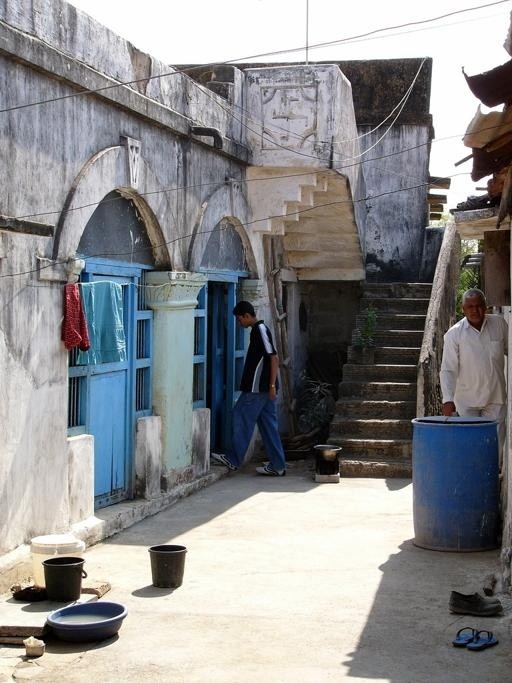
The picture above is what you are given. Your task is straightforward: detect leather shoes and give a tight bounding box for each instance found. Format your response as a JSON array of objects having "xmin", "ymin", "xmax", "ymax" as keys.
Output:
[
  {"xmin": 450, "ymin": 591, "xmax": 500, "ymax": 605},
  {"xmin": 449, "ymin": 598, "xmax": 502, "ymax": 617}
]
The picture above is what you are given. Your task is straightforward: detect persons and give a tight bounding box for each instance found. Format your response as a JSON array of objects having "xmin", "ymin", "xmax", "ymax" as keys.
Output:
[
  {"xmin": 212, "ymin": 300, "xmax": 286, "ymax": 476},
  {"xmin": 439, "ymin": 287, "xmax": 508, "ymax": 473},
  {"xmin": 307, "ymin": 343, "xmax": 345, "ymax": 382}
]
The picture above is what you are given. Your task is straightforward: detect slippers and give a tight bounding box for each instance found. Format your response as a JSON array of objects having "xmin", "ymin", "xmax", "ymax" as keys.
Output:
[
  {"xmin": 466, "ymin": 630, "xmax": 499, "ymax": 651},
  {"xmin": 451, "ymin": 626, "xmax": 479, "ymax": 646}
]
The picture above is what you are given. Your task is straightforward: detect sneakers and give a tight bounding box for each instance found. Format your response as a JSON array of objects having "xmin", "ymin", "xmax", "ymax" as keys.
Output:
[
  {"xmin": 211, "ymin": 452, "xmax": 239, "ymax": 471},
  {"xmin": 255, "ymin": 464, "xmax": 286, "ymax": 477}
]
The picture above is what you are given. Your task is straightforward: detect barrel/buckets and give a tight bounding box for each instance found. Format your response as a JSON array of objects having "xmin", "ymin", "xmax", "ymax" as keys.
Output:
[
  {"xmin": 313, "ymin": 445, "xmax": 342, "ymax": 475},
  {"xmin": 412, "ymin": 415, "xmax": 500, "ymax": 553},
  {"xmin": 30, "ymin": 535, "xmax": 86, "ymax": 589},
  {"xmin": 41, "ymin": 557, "xmax": 85, "ymax": 602},
  {"xmin": 148, "ymin": 544, "xmax": 186, "ymax": 588}
]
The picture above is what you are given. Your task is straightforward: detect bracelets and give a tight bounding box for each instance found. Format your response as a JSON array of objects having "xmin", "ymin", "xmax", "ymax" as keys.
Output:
[{"xmin": 269, "ymin": 383, "xmax": 275, "ymax": 387}]
